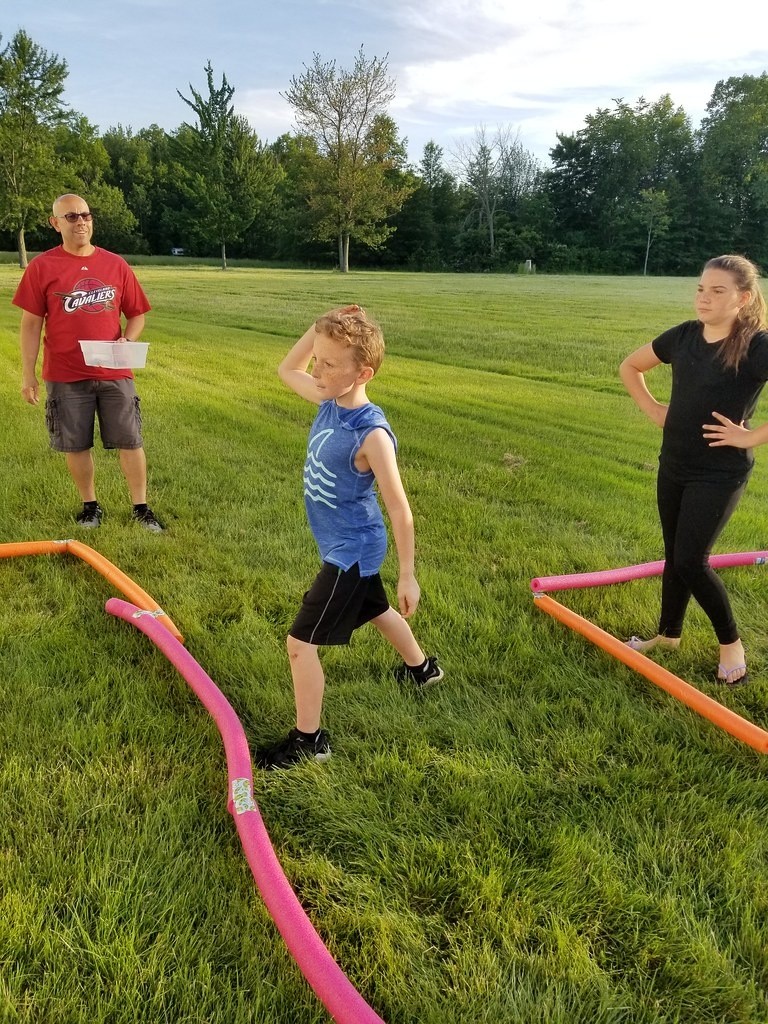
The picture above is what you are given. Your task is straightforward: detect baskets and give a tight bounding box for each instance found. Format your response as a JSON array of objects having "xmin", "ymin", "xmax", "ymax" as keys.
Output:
[{"xmin": 79, "ymin": 340, "xmax": 149, "ymax": 369}]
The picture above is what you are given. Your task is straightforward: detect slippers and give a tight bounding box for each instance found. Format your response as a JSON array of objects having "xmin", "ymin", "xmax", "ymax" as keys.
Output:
[
  {"xmin": 716, "ymin": 655, "xmax": 747, "ymax": 687},
  {"xmin": 628, "ymin": 636, "xmax": 644, "ymax": 651}
]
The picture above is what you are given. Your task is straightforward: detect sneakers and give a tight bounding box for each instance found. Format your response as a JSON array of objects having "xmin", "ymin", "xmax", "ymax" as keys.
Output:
[
  {"xmin": 132, "ymin": 509, "xmax": 163, "ymax": 533},
  {"xmin": 403, "ymin": 656, "xmax": 444, "ymax": 690},
  {"xmin": 76, "ymin": 506, "xmax": 102, "ymax": 528},
  {"xmin": 253, "ymin": 729, "xmax": 331, "ymax": 773}
]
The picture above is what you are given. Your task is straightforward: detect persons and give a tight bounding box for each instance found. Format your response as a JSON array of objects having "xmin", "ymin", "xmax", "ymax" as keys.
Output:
[
  {"xmin": 618, "ymin": 253, "xmax": 767, "ymax": 686},
  {"xmin": 13, "ymin": 194, "xmax": 166, "ymax": 536},
  {"xmin": 255, "ymin": 306, "xmax": 444, "ymax": 772}
]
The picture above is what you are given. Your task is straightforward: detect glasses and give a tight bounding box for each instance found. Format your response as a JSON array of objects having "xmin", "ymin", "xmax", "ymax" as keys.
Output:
[{"xmin": 56, "ymin": 213, "xmax": 94, "ymax": 223}]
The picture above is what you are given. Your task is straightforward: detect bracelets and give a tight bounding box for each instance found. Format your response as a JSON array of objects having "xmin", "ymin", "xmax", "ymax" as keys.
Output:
[{"xmin": 126, "ymin": 338, "xmax": 131, "ymax": 342}]
